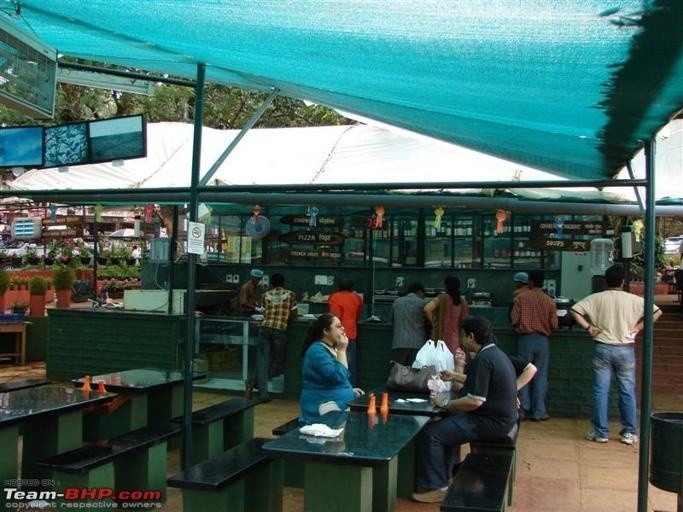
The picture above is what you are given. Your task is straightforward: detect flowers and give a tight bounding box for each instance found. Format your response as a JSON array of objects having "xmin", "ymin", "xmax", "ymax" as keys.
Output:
[{"xmin": 60, "ymin": 242, "xmax": 72, "ymax": 261}]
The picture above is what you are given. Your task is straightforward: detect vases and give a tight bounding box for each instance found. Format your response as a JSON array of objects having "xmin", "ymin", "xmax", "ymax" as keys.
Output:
[{"xmin": 59, "ymin": 257, "xmax": 72, "ymax": 265}]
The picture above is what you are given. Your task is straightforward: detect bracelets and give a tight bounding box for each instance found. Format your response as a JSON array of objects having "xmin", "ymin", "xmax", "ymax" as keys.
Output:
[{"xmin": 585, "ymin": 324, "xmax": 591, "ymax": 330}]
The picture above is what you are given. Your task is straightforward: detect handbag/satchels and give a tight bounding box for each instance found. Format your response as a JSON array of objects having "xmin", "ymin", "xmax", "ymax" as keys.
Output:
[
  {"xmin": 385, "ymin": 360, "xmax": 437, "ymax": 396},
  {"xmin": 430, "ymin": 295, "xmax": 446, "ymax": 344}
]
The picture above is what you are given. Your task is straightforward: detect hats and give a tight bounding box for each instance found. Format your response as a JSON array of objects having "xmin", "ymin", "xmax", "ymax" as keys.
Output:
[
  {"xmin": 250, "ymin": 269, "xmax": 264, "ymax": 278},
  {"xmin": 514, "ymin": 272, "xmax": 529, "ymax": 283}
]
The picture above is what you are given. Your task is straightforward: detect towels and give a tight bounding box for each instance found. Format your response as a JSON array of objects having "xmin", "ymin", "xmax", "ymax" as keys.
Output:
[{"xmin": 300, "ymin": 423, "xmax": 343, "ymax": 437}]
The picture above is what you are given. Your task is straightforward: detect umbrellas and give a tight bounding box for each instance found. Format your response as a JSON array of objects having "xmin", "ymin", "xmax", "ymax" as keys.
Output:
[{"xmin": 109, "ymin": 228, "xmax": 144, "ymax": 241}]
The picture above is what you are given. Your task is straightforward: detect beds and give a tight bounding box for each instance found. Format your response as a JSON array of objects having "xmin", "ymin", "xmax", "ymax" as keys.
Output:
[
  {"xmin": 346, "ymin": 381, "xmax": 444, "ymax": 500},
  {"xmin": 72, "ymin": 367, "xmax": 207, "ymax": 443}
]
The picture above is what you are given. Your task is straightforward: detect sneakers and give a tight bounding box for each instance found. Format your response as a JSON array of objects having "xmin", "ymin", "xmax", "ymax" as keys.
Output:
[
  {"xmin": 620, "ymin": 433, "xmax": 639, "ymax": 444},
  {"xmin": 245, "ymin": 378, "xmax": 254, "ymax": 400},
  {"xmin": 411, "ymin": 490, "xmax": 448, "ymax": 503},
  {"xmin": 584, "ymin": 430, "xmax": 608, "ymax": 442},
  {"xmin": 519, "ymin": 412, "xmax": 528, "ymax": 420},
  {"xmin": 529, "ymin": 414, "xmax": 550, "ymax": 422},
  {"xmin": 446, "ymin": 479, "xmax": 453, "ymax": 487},
  {"xmin": 258, "ymin": 396, "xmax": 273, "ymax": 404}
]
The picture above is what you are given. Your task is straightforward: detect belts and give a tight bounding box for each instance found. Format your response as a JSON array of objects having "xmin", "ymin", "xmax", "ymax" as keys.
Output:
[
  {"xmin": 592, "ymin": 340, "xmax": 634, "ymax": 347},
  {"xmin": 521, "ymin": 332, "xmax": 544, "ymax": 335}
]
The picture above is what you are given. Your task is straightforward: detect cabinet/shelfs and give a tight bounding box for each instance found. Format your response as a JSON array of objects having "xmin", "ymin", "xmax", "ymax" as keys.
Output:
[{"xmin": 285, "ymin": 214, "xmax": 566, "ymax": 273}]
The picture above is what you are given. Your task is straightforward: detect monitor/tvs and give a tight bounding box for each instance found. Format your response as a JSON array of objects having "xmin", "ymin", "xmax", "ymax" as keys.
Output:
[
  {"xmin": 0, "ymin": 126, "xmax": 46, "ymax": 168},
  {"xmin": 38, "ymin": 121, "xmax": 88, "ymax": 169},
  {"xmin": 88, "ymin": 114, "xmax": 147, "ymax": 163}
]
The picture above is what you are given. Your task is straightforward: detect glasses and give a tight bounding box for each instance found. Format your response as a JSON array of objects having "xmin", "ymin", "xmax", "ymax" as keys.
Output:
[{"xmin": 254, "ymin": 278, "xmax": 260, "ymax": 281}]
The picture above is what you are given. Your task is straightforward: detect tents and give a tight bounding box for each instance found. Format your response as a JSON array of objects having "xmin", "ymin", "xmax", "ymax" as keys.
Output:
[
  {"xmin": 3, "ymin": 118, "xmax": 683, "ymax": 299},
  {"xmin": 0, "ymin": 2, "xmax": 682, "ymax": 512}
]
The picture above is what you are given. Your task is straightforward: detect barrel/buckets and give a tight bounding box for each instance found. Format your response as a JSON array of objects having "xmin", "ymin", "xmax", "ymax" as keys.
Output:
[{"xmin": 590, "ymin": 238, "xmax": 614, "ymax": 275}]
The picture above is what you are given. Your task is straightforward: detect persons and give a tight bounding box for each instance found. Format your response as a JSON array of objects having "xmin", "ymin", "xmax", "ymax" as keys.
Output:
[
  {"xmin": 512, "ymin": 272, "xmax": 529, "ymax": 299},
  {"xmin": 388, "ymin": 283, "xmax": 425, "ymax": 364},
  {"xmin": 410, "ymin": 314, "xmax": 517, "ymax": 503},
  {"xmin": 327, "ymin": 279, "xmax": 362, "ymax": 386},
  {"xmin": 131, "ymin": 245, "xmax": 140, "ymax": 267},
  {"xmin": 257, "ymin": 273, "xmax": 297, "ymax": 403},
  {"xmin": 239, "ymin": 269, "xmax": 264, "ymax": 314},
  {"xmin": 300, "ymin": 312, "xmax": 365, "ymax": 424},
  {"xmin": 569, "ymin": 265, "xmax": 662, "ymax": 446},
  {"xmin": 424, "ymin": 274, "xmax": 468, "ymax": 355},
  {"xmin": 510, "ymin": 272, "xmax": 557, "ymax": 422},
  {"xmin": 451, "ymin": 331, "xmax": 538, "ymax": 410}
]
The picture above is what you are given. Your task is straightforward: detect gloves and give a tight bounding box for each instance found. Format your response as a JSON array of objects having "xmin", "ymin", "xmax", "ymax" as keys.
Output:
[{"xmin": 255, "ymin": 307, "xmax": 265, "ymax": 313}]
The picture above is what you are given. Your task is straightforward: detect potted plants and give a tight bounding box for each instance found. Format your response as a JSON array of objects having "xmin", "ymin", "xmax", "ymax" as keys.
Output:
[
  {"xmin": 29, "ymin": 275, "xmax": 48, "ymax": 316},
  {"xmin": 94, "ymin": 266, "xmax": 142, "ymax": 292},
  {"xmin": 125, "ymin": 247, "xmax": 137, "ymax": 265},
  {"xmin": 27, "ymin": 248, "xmax": 40, "ymax": 265},
  {"xmin": 54, "ymin": 267, "xmax": 75, "ymax": 308},
  {"xmin": 0, "ymin": 270, "xmax": 11, "ymax": 314},
  {"xmin": 97, "ymin": 246, "xmax": 108, "ymax": 265},
  {"xmin": 77, "ymin": 239, "xmax": 92, "ymax": 265},
  {"xmin": 7, "ymin": 301, "xmax": 28, "ymax": 314},
  {"xmin": 71, "ymin": 281, "xmax": 90, "ymax": 309},
  {"xmin": 43, "ymin": 239, "xmax": 58, "ymax": 265},
  {"xmin": 110, "ymin": 245, "xmax": 123, "ymax": 265}
]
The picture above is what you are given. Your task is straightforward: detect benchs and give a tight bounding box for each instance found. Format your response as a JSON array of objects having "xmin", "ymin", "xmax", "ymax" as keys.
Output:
[
  {"xmin": 470, "ymin": 422, "xmax": 519, "ymax": 445},
  {"xmin": 169, "ymin": 398, "xmax": 264, "ymax": 471},
  {"xmin": 440, "ymin": 451, "xmax": 515, "ymax": 511},
  {"xmin": 272, "ymin": 417, "xmax": 313, "ymax": 489},
  {"xmin": 0, "ymin": 379, "xmax": 51, "ymax": 394},
  {"xmin": 34, "ymin": 422, "xmax": 182, "ymax": 512},
  {"xmin": 167, "ymin": 437, "xmax": 282, "ymax": 512}
]
